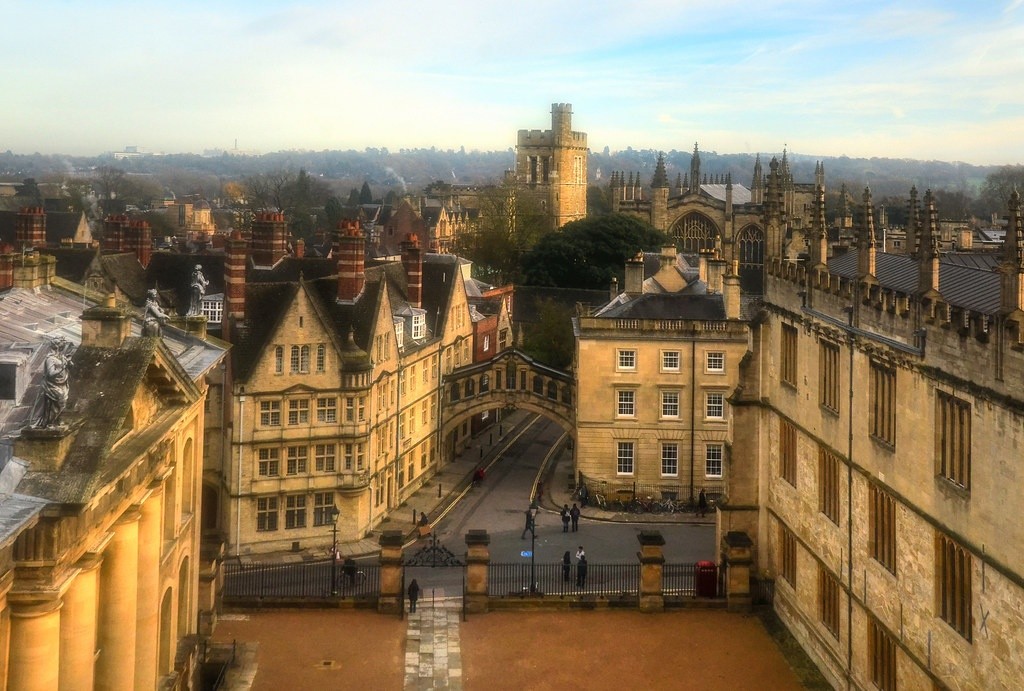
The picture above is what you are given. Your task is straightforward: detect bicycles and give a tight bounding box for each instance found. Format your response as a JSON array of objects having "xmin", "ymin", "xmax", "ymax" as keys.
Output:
[
  {"xmin": 571, "ymin": 484, "xmax": 718, "ymax": 514},
  {"xmin": 335, "ymin": 570, "xmax": 367, "ymax": 592}
]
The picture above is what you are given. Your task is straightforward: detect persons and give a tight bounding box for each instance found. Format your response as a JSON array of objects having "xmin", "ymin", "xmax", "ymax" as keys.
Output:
[
  {"xmin": 696, "ymin": 488, "xmax": 707, "ymax": 518},
  {"xmin": 521, "ymin": 509, "xmax": 538, "ymax": 539},
  {"xmin": 575, "ymin": 546, "xmax": 585, "ymax": 563},
  {"xmin": 569, "ymin": 503, "xmax": 580, "ymax": 532},
  {"xmin": 560, "ymin": 504, "xmax": 571, "ymax": 533},
  {"xmin": 416, "ymin": 512, "xmax": 428, "ymax": 527},
  {"xmin": 471, "ymin": 466, "xmax": 485, "ymax": 488},
  {"xmin": 340, "ymin": 558, "xmax": 358, "ymax": 589},
  {"xmin": 407, "ymin": 579, "xmax": 419, "ymax": 614},
  {"xmin": 576, "ymin": 554, "xmax": 588, "ymax": 586},
  {"xmin": 562, "ymin": 551, "xmax": 571, "ymax": 582}
]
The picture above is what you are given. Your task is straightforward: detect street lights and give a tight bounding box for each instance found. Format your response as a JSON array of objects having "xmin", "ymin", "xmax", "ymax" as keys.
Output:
[
  {"xmin": 330, "ymin": 502, "xmax": 341, "ymax": 594},
  {"xmin": 529, "ymin": 499, "xmax": 540, "ymax": 593}
]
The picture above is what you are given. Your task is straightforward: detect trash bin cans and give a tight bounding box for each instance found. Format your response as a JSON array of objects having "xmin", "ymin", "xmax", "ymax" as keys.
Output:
[{"xmin": 694, "ymin": 560, "xmax": 717, "ymax": 598}]
